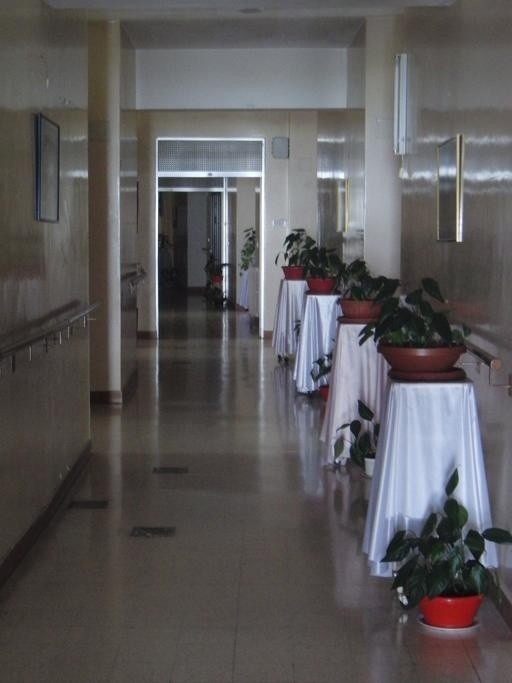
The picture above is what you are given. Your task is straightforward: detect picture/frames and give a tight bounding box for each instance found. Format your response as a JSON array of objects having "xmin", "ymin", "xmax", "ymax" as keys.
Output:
[
  {"xmin": 436, "ymin": 134, "xmax": 463, "ymax": 243},
  {"xmin": 34, "ymin": 112, "xmax": 60, "ymax": 223}
]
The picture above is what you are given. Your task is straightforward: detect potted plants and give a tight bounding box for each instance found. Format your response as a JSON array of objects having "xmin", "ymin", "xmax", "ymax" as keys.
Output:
[
  {"xmin": 311, "ymin": 339, "xmax": 334, "ymax": 401},
  {"xmin": 334, "ymin": 399, "xmax": 381, "ymax": 479},
  {"xmin": 376, "ymin": 466, "xmax": 512, "ymax": 628},
  {"xmin": 272, "ymin": 227, "xmax": 466, "ymax": 382},
  {"xmin": 202, "ymin": 245, "xmax": 234, "ymax": 283}
]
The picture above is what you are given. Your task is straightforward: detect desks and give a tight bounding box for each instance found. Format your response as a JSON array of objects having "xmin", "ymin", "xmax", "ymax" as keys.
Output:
[
  {"xmin": 318, "ymin": 323, "xmax": 396, "ymax": 467},
  {"xmin": 360, "ymin": 380, "xmax": 498, "ymax": 580}
]
